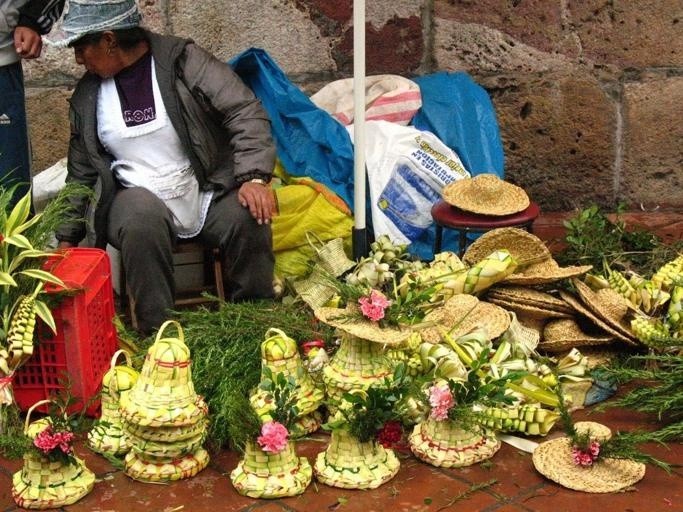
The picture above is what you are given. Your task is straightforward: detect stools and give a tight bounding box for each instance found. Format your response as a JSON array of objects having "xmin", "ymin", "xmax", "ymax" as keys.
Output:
[
  {"xmin": 118, "ymin": 239, "xmax": 226, "ymax": 332},
  {"xmin": 429, "ymin": 200, "xmax": 540, "ymax": 261}
]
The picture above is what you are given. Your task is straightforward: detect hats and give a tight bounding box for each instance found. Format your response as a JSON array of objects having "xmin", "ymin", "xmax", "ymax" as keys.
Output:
[
  {"xmin": 442, "ymin": 173, "xmax": 531, "ymax": 216},
  {"xmin": 37, "ymin": 0, "xmax": 146, "ymax": 47},
  {"xmin": 531, "ymin": 420, "xmax": 647, "ymax": 494},
  {"xmin": 310, "ymin": 226, "xmax": 667, "ymax": 371}
]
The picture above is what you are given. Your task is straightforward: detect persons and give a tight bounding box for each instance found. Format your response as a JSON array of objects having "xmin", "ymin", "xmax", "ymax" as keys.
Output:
[
  {"xmin": 48, "ymin": 0, "xmax": 282, "ymax": 344},
  {"xmin": 0, "ymin": 0, "xmax": 68, "ymax": 234}
]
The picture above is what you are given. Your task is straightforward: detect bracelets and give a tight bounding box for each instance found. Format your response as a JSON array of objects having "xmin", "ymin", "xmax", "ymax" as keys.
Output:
[{"xmin": 248, "ymin": 178, "xmax": 266, "ymax": 186}]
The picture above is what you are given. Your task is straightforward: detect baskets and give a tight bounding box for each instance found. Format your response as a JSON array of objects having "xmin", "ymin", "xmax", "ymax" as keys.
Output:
[{"xmin": 11, "ymin": 246, "xmax": 120, "ymax": 420}]
